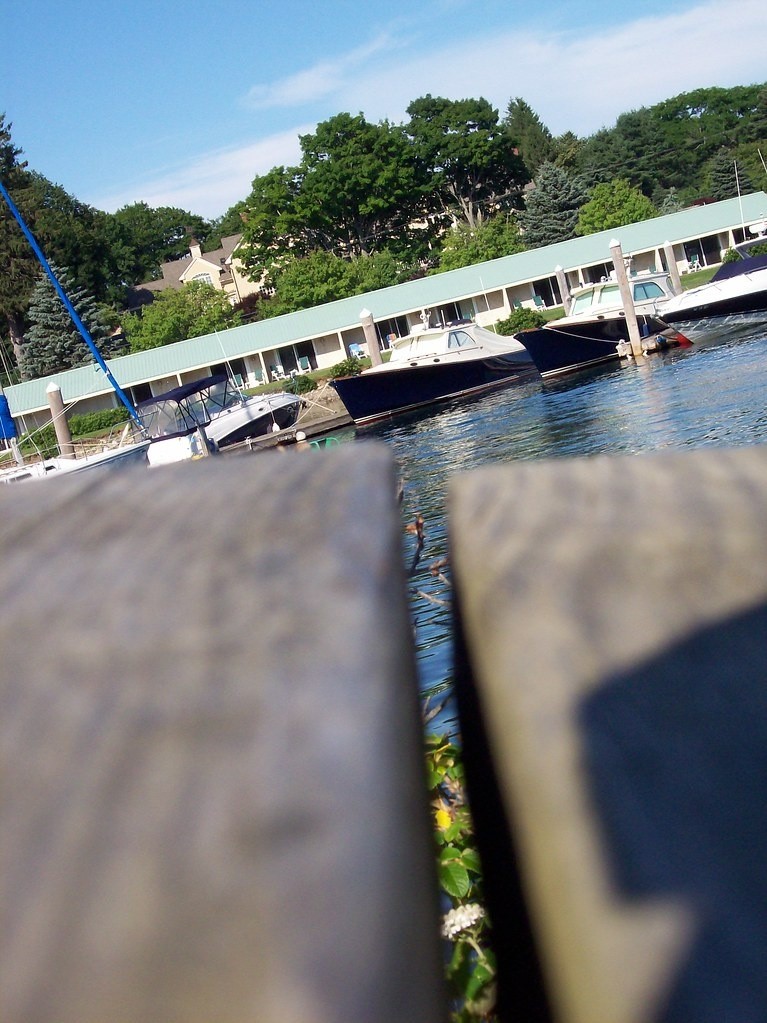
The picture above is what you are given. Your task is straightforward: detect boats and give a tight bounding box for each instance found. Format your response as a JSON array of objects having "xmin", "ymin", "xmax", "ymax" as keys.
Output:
[
  {"xmin": 656, "ymin": 160, "xmax": 767, "ymax": 323},
  {"xmin": 513, "ymin": 259, "xmax": 682, "ymax": 378},
  {"xmin": 134, "ymin": 329, "xmax": 304, "ymax": 469},
  {"xmin": 326, "ymin": 274, "xmax": 538, "ymax": 428}
]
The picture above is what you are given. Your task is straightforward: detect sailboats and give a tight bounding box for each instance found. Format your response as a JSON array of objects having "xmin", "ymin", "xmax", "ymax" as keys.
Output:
[{"xmin": 0, "ymin": 184, "xmax": 157, "ymax": 482}]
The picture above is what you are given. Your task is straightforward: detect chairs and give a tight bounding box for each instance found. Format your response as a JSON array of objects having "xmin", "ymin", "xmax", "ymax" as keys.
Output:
[
  {"xmin": 298, "ymin": 356, "xmax": 312, "ymax": 375},
  {"xmin": 387, "ymin": 333, "xmax": 397, "ymax": 348},
  {"xmin": 234, "ymin": 373, "xmax": 250, "ymax": 391},
  {"xmin": 532, "ymin": 294, "xmax": 548, "ymax": 312},
  {"xmin": 629, "ymin": 268, "xmax": 637, "ymax": 277},
  {"xmin": 688, "ymin": 253, "xmax": 703, "ymax": 273},
  {"xmin": 648, "ymin": 264, "xmax": 657, "ymax": 272},
  {"xmin": 512, "ymin": 298, "xmax": 523, "ymax": 311},
  {"xmin": 254, "ymin": 366, "xmax": 265, "ymax": 385},
  {"xmin": 348, "ymin": 343, "xmax": 368, "ymax": 360},
  {"xmin": 269, "ymin": 363, "xmax": 286, "ymax": 381}
]
